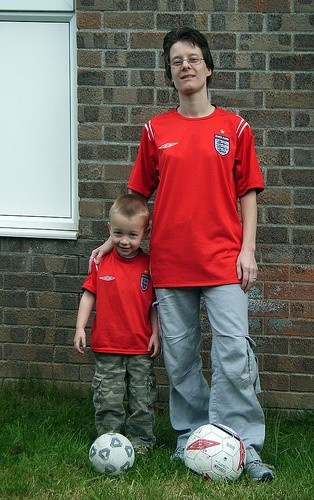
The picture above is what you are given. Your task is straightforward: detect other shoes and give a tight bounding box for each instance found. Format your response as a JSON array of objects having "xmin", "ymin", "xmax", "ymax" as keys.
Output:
[{"xmin": 134, "ymin": 445, "xmax": 149, "ymax": 456}]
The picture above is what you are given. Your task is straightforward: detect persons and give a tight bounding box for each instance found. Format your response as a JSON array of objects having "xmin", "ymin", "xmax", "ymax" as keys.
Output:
[
  {"xmin": 88, "ymin": 27, "xmax": 274, "ymax": 481},
  {"xmin": 73, "ymin": 195, "xmax": 160, "ymax": 457}
]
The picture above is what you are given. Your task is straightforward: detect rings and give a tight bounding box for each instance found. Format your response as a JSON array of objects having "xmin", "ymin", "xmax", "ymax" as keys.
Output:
[{"xmin": 254, "ymin": 277, "xmax": 256, "ymax": 279}]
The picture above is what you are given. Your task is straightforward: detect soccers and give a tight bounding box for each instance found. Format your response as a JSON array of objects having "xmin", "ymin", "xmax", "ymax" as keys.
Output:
[
  {"xmin": 183, "ymin": 422, "xmax": 245, "ymax": 483},
  {"xmin": 87, "ymin": 432, "xmax": 135, "ymax": 476}
]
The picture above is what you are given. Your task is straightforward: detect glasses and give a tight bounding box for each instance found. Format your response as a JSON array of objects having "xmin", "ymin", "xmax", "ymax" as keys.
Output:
[{"xmin": 168, "ymin": 57, "xmax": 204, "ymax": 67}]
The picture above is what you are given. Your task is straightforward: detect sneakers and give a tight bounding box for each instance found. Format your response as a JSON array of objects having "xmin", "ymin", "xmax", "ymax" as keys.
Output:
[
  {"xmin": 242, "ymin": 460, "xmax": 275, "ymax": 482},
  {"xmin": 169, "ymin": 446, "xmax": 184, "ymax": 463}
]
